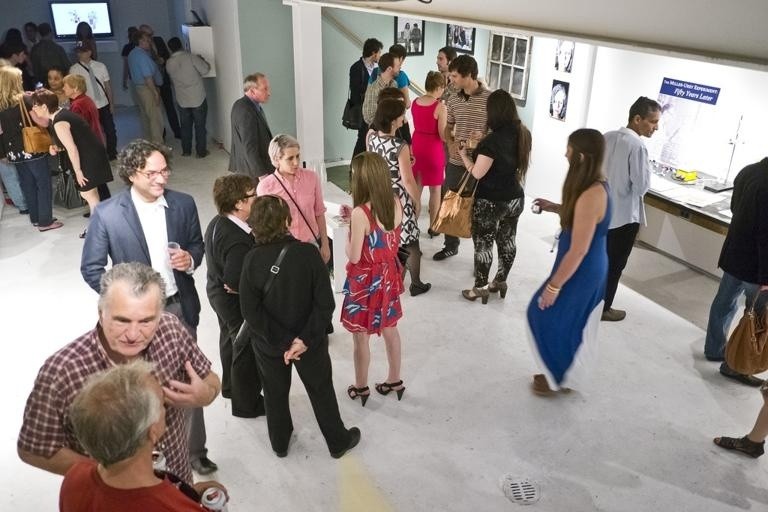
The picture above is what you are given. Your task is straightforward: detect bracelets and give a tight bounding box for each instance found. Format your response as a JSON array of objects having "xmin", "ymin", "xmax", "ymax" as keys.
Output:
[{"xmin": 545, "ymin": 280, "xmax": 560, "ymax": 295}]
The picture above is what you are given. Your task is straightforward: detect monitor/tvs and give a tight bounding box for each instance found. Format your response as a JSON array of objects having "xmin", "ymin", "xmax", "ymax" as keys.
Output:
[{"xmin": 49, "ymin": 1, "xmax": 114, "ymax": 40}]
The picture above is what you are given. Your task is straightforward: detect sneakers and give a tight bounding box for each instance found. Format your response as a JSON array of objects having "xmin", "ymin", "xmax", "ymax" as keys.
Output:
[{"xmin": 433, "ymin": 247, "xmax": 458, "ymax": 261}]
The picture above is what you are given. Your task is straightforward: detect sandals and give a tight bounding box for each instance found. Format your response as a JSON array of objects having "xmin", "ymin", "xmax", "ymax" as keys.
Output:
[{"xmin": 714, "ymin": 434, "xmax": 766, "ymax": 458}]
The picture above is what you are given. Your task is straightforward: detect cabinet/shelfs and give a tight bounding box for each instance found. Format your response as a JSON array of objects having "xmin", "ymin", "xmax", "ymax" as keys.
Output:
[{"xmin": 182, "ymin": 23, "xmax": 216, "ymax": 78}]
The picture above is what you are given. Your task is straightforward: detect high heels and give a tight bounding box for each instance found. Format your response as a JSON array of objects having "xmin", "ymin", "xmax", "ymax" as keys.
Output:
[
  {"xmin": 462, "ymin": 286, "xmax": 489, "ymax": 304},
  {"xmin": 428, "ymin": 228, "xmax": 439, "ymax": 238},
  {"xmin": 375, "ymin": 379, "xmax": 405, "ymax": 402},
  {"xmin": 348, "ymin": 386, "xmax": 370, "ymax": 408},
  {"xmin": 488, "ymin": 281, "xmax": 507, "ymax": 298}
]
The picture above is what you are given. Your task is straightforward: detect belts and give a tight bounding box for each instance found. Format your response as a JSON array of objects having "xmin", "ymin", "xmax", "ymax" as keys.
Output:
[{"xmin": 167, "ymin": 291, "xmax": 180, "ymax": 305}]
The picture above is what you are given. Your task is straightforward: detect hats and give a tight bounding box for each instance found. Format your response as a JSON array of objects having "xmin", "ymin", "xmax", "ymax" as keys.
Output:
[{"xmin": 71, "ymin": 41, "xmax": 92, "ymax": 52}]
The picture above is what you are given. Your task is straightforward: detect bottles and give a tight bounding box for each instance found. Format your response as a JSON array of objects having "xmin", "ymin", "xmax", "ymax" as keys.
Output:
[{"xmin": 152, "ymin": 450, "xmax": 166, "ymax": 470}]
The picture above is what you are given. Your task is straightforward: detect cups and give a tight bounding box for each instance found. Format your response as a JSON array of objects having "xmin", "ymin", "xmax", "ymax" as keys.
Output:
[
  {"xmin": 147, "ymin": 450, "xmax": 170, "ymax": 473},
  {"xmin": 469, "ymin": 130, "xmax": 481, "ymax": 148},
  {"xmin": 531, "ymin": 200, "xmax": 541, "ymax": 216},
  {"xmin": 163, "ymin": 242, "xmax": 181, "ymax": 270},
  {"xmin": 326, "ymin": 205, "xmax": 346, "ymax": 218},
  {"xmin": 695, "ymin": 177, "xmax": 705, "ymax": 192}
]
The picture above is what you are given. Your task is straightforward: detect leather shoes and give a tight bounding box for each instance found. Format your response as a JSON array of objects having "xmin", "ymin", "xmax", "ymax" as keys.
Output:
[
  {"xmin": 38, "ymin": 223, "xmax": 63, "ymax": 232},
  {"xmin": 197, "ymin": 150, "xmax": 208, "ymax": 158},
  {"xmin": 409, "ymin": 283, "xmax": 431, "ymax": 296},
  {"xmin": 182, "ymin": 151, "xmax": 191, "ymax": 156},
  {"xmin": 600, "ymin": 307, "xmax": 626, "ymax": 320},
  {"xmin": 175, "ymin": 133, "xmax": 181, "ymax": 138},
  {"xmin": 51, "ymin": 216, "xmax": 57, "ymax": 221},
  {"xmin": 80, "ymin": 229, "xmax": 87, "ymax": 238},
  {"xmin": 721, "ymin": 362, "xmax": 765, "ymax": 388},
  {"xmin": 191, "ymin": 456, "xmax": 217, "ymax": 474},
  {"xmin": 705, "ymin": 355, "xmax": 724, "ymax": 361},
  {"xmin": 20, "ymin": 210, "xmax": 28, "ymax": 214},
  {"xmin": 84, "ymin": 212, "xmax": 90, "ymax": 217},
  {"xmin": 332, "ymin": 425, "xmax": 361, "ymax": 459},
  {"xmin": 276, "ymin": 450, "xmax": 287, "ymax": 457},
  {"xmin": 532, "ymin": 373, "xmax": 571, "ymax": 396}
]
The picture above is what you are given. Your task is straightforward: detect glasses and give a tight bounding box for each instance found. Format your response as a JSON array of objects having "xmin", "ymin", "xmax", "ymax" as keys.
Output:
[
  {"xmin": 132, "ymin": 166, "xmax": 172, "ymax": 179},
  {"xmin": 243, "ymin": 192, "xmax": 257, "ymax": 200}
]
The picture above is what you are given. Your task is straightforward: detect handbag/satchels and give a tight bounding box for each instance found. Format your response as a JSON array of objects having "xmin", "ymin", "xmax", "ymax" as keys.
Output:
[
  {"xmin": 77, "ymin": 60, "xmax": 110, "ymax": 104},
  {"xmin": 18, "ymin": 94, "xmax": 52, "ymax": 154},
  {"xmin": 724, "ymin": 288, "xmax": 768, "ymax": 375},
  {"xmin": 54, "ymin": 152, "xmax": 86, "ymax": 209},
  {"xmin": 211, "ymin": 217, "xmax": 223, "ymax": 259},
  {"xmin": 272, "ymin": 172, "xmax": 332, "ymax": 276},
  {"xmin": 431, "ymin": 163, "xmax": 480, "ymax": 239},
  {"xmin": 342, "ymin": 61, "xmax": 365, "ymax": 131},
  {"xmin": 258, "ymin": 242, "xmax": 290, "ymax": 308}
]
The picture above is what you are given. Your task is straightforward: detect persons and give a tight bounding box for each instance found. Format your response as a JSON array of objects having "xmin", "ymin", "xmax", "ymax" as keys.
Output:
[
  {"xmin": 75, "ymin": 22, "xmax": 97, "ymax": 62},
  {"xmin": 458, "ymin": 89, "xmax": 532, "ymax": 305},
  {"xmin": 140, "ymin": 25, "xmax": 182, "ymax": 140},
  {"xmin": 338, "ymin": 39, "xmax": 385, "ymax": 191},
  {"xmin": 705, "ymin": 156, "xmax": 768, "ymax": 387},
  {"xmin": 714, "ymin": 381, "xmax": 767, "ymax": 458},
  {"xmin": 0, "ymin": 66, "xmax": 64, "ymax": 232},
  {"xmin": 401, "ymin": 22, "xmax": 423, "ymax": 54},
  {"xmin": 227, "ymin": 74, "xmax": 275, "ymax": 185},
  {"xmin": 411, "ymin": 71, "xmax": 451, "ymax": 239},
  {"xmin": 25, "ymin": 23, "xmax": 41, "ymax": 47},
  {"xmin": 0, "ymin": 28, "xmax": 34, "ymax": 90},
  {"xmin": 436, "ymin": 48, "xmax": 457, "ymax": 104},
  {"xmin": 121, "ymin": 27, "xmax": 139, "ymax": 89},
  {"xmin": 0, "ymin": 43, "xmax": 28, "ymax": 66},
  {"xmin": 204, "ymin": 175, "xmax": 267, "ymax": 420},
  {"xmin": 16, "ymin": 262, "xmax": 221, "ymax": 486},
  {"xmin": 527, "ymin": 128, "xmax": 610, "ymax": 398},
  {"xmin": 432, "ymin": 55, "xmax": 493, "ymax": 262},
  {"xmin": 551, "ymin": 84, "xmax": 567, "ymax": 121},
  {"xmin": 362, "ymin": 53, "xmax": 400, "ymax": 128},
  {"xmin": 44, "ymin": 68, "xmax": 70, "ymax": 106},
  {"xmin": 555, "ymin": 41, "xmax": 574, "ymax": 73},
  {"xmin": 58, "ymin": 363, "xmax": 230, "ymax": 510},
  {"xmin": 239, "ymin": 194, "xmax": 361, "ymax": 459},
  {"xmin": 367, "ymin": 44, "xmax": 411, "ymax": 139},
  {"xmin": 68, "ymin": 39, "xmax": 118, "ymax": 160},
  {"xmin": 339, "ymin": 150, "xmax": 404, "ymax": 407},
  {"xmin": 366, "ymin": 95, "xmax": 432, "ymax": 297},
  {"xmin": 127, "ymin": 31, "xmax": 173, "ymax": 151},
  {"xmin": 165, "ymin": 38, "xmax": 210, "ymax": 158},
  {"xmin": 82, "ymin": 140, "xmax": 219, "ymax": 477},
  {"xmin": 601, "ymin": 98, "xmax": 663, "ymax": 322},
  {"xmin": 446, "ymin": 25, "xmax": 474, "ymax": 54},
  {"xmin": 378, "ymin": 88, "xmax": 404, "ymax": 100},
  {"xmin": 31, "ymin": 23, "xmax": 70, "ymax": 84},
  {"xmin": 62, "ymin": 74, "xmax": 111, "ymax": 217},
  {"xmin": 253, "ymin": 134, "xmax": 331, "ymax": 274},
  {"xmin": 31, "ymin": 89, "xmax": 113, "ymax": 237}
]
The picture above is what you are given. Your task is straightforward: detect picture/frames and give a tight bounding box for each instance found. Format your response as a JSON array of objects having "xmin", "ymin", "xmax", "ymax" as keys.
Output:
[
  {"xmin": 393, "ymin": 16, "xmax": 425, "ymax": 56},
  {"xmin": 445, "ymin": 23, "xmax": 476, "ymax": 56},
  {"xmin": 554, "ymin": 39, "xmax": 575, "ymax": 73},
  {"xmin": 549, "ymin": 79, "xmax": 570, "ymax": 122}
]
